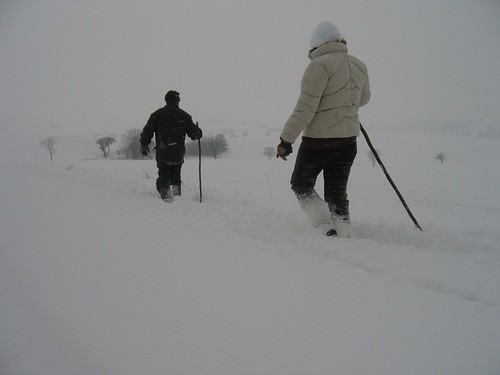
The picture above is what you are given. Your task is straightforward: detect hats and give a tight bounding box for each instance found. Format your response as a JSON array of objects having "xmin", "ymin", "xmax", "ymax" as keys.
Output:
[{"xmin": 310, "ymin": 21, "xmax": 342, "ymax": 48}]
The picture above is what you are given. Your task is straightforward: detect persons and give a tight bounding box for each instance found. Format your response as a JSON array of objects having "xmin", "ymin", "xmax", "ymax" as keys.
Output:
[
  {"xmin": 140, "ymin": 90, "xmax": 202, "ymax": 203},
  {"xmin": 276, "ymin": 21, "xmax": 371, "ymax": 237}
]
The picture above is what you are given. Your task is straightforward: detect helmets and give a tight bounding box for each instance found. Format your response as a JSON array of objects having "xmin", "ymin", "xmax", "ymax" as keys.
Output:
[{"xmin": 165, "ymin": 90, "xmax": 180, "ymax": 106}]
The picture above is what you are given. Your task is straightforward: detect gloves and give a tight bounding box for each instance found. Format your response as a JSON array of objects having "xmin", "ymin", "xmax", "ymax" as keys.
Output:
[
  {"xmin": 140, "ymin": 143, "xmax": 150, "ymax": 156},
  {"xmin": 275, "ymin": 138, "xmax": 294, "ymax": 161},
  {"xmin": 195, "ymin": 128, "xmax": 203, "ymax": 139}
]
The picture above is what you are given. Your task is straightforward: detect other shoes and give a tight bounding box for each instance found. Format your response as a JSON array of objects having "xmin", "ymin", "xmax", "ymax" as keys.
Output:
[{"xmin": 325, "ymin": 229, "xmax": 337, "ymax": 238}]
